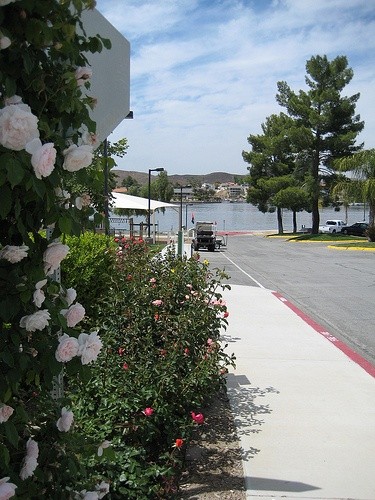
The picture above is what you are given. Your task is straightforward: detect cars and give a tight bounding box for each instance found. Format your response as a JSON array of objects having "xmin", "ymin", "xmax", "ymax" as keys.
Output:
[{"xmin": 341, "ymin": 221, "xmax": 372, "ymax": 235}]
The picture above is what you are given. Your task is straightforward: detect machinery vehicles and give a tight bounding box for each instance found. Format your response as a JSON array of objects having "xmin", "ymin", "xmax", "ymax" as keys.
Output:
[{"xmin": 189, "ymin": 221, "xmax": 229, "ymax": 252}]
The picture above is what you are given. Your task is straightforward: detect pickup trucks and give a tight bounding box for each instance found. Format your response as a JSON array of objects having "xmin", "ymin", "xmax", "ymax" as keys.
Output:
[{"xmin": 318, "ymin": 219, "xmax": 348, "ymax": 234}]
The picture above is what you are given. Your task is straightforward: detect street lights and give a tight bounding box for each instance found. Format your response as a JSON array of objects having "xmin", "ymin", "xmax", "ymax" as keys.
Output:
[
  {"xmin": 179, "ymin": 185, "xmax": 192, "ymax": 234},
  {"xmin": 148, "ymin": 167, "xmax": 164, "ymax": 239},
  {"xmin": 185, "ymin": 203, "xmax": 193, "ymax": 231},
  {"xmin": 103, "ymin": 110, "xmax": 134, "ymax": 236}
]
